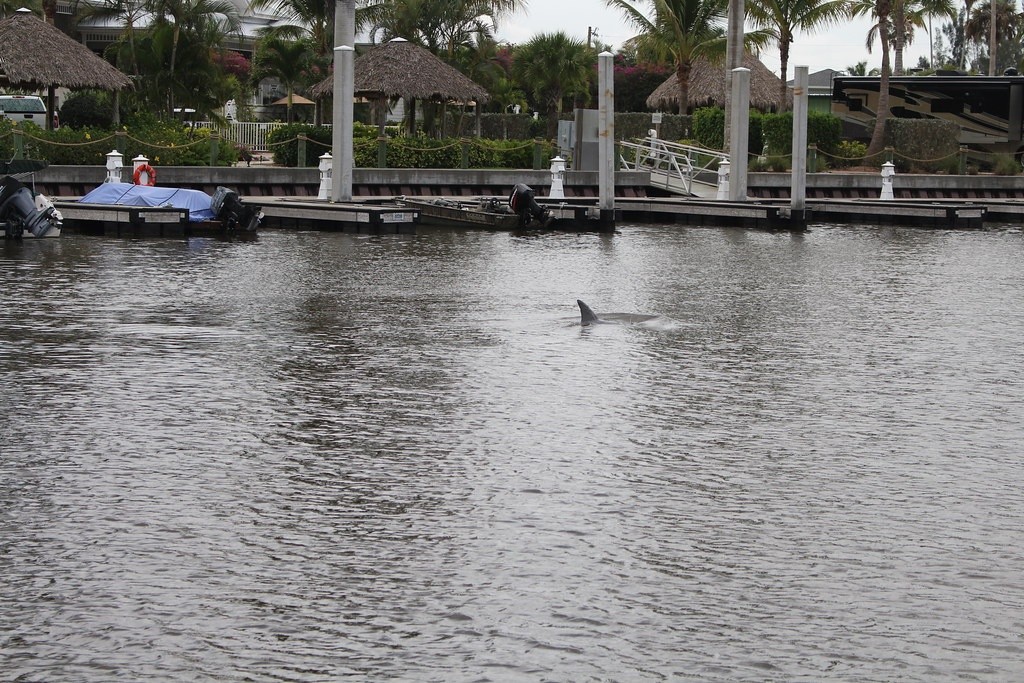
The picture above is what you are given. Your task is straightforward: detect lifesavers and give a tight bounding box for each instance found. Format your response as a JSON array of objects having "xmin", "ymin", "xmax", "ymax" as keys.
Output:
[{"xmin": 133, "ymin": 164, "xmax": 156, "ymax": 187}]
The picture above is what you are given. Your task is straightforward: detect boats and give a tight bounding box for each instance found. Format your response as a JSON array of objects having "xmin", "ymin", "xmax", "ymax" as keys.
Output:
[
  {"xmin": 0, "ymin": 157, "xmax": 64, "ymax": 239},
  {"xmin": 392, "ymin": 182, "xmax": 555, "ymax": 229},
  {"xmin": 44, "ymin": 182, "xmax": 264, "ymax": 238}
]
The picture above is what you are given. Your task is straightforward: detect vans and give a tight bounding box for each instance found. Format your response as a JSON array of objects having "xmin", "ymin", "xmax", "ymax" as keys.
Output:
[{"xmin": 0, "ymin": 95, "xmax": 48, "ymax": 131}]
